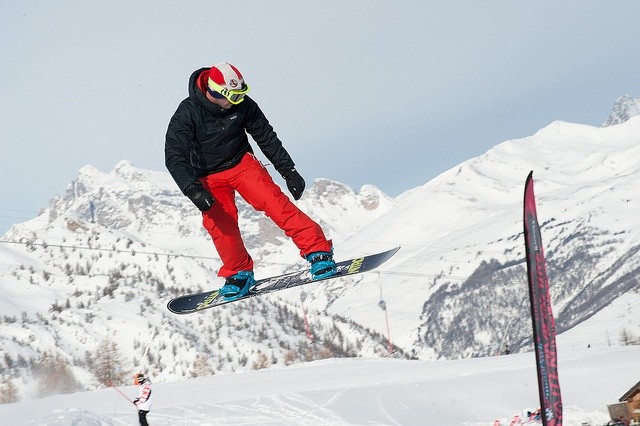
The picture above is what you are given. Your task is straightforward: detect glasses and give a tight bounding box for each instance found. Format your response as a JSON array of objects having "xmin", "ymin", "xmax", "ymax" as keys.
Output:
[{"xmin": 208, "ymin": 77, "xmax": 250, "ymax": 105}]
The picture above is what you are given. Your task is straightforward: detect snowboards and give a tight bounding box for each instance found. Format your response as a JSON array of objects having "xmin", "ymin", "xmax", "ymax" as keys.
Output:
[{"xmin": 166, "ymin": 247, "xmax": 401, "ymax": 315}]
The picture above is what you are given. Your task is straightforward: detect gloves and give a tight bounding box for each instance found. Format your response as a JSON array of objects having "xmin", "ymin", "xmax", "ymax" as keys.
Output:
[
  {"xmin": 185, "ymin": 180, "xmax": 215, "ymax": 211},
  {"xmin": 283, "ymin": 167, "xmax": 306, "ymax": 201}
]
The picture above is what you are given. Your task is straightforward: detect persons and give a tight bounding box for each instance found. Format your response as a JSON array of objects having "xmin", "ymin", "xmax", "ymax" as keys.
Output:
[
  {"xmin": 133, "ymin": 374, "xmax": 152, "ymax": 426},
  {"xmin": 164, "ymin": 62, "xmax": 338, "ymax": 302}
]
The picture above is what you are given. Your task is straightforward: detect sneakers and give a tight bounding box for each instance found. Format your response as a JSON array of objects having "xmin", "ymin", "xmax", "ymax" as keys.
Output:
[
  {"xmin": 218, "ymin": 270, "xmax": 257, "ymax": 302},
  {"xmin": 306, "ymin": 245, "xmax": 337, "ymax": 281}
]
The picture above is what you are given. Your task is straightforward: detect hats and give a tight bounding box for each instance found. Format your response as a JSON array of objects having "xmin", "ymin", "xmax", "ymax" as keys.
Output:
[{"xmin": 208, "ymin": 61, "xmax": 245, "ymax": 99}]
